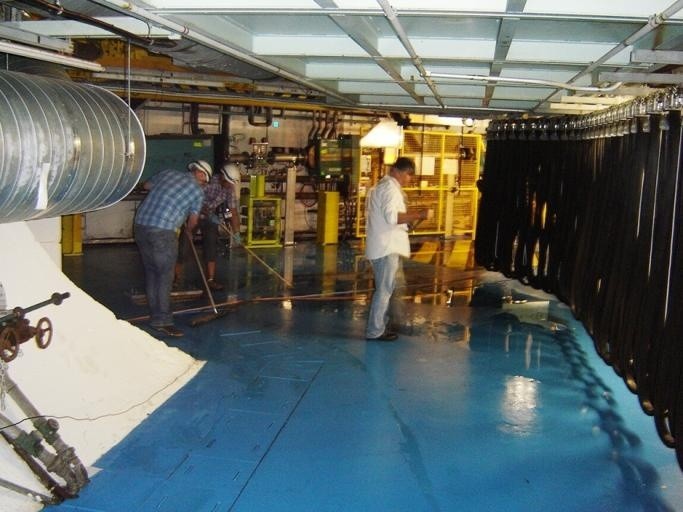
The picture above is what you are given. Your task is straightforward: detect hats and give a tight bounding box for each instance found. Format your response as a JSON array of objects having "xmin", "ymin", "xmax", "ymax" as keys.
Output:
[
  {"xmin": 381, "ymin": 156, "xmax": 416, "ymax": 173},
  {"xmin": 188, "ymin": 161, "xmax": 212, "ymax": 183},
  {"xmin": 219, "ymin": 163, "xmax": 242, "ymax": 184}
]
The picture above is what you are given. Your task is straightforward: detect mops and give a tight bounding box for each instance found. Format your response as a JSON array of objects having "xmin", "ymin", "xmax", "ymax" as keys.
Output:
[{"xmin": 182, "ymin": 222, "xmax": 231, "ymax": 329}]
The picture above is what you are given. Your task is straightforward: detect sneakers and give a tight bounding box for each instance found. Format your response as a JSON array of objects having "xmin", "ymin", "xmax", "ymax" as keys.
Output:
[
  {"xmin": 203, "ymin": 275, "xmax": 225, "ymax": 291},
  {"xmin": 390, "ymin": 322, "xmax": 420, "ymax": 334},
  {"xmin": 151, "ymin": 323, "xmax": 185, "ymax": 337},
  {"xmin": 367, "ymin": 331, "xmax": 397, "ymax": 341}
]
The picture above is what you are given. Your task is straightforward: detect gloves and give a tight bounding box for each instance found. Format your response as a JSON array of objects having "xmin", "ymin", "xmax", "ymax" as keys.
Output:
[
  {"xmin": 207, "ymin": 213, "xmax": 220, "ymax": 225},
  {"xmin": 233, "ymin": 232, "xmax": 241, "ymax": 246}
]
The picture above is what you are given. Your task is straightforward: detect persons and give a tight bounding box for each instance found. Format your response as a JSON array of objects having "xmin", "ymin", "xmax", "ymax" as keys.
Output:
[
  {"xmin": 132, "ymin": 158, "xmax": 213, "ymax": 336},
  {"xmin": 172, "ymin": 163, "xmax": 241, "ymax": 291},
  {"xmin": 365, "ymin": 157, "xmax": 427, "ymax": 341}
]
